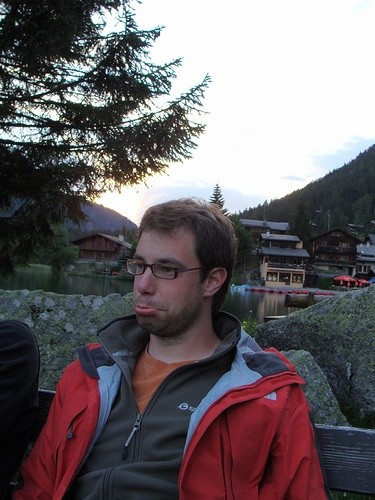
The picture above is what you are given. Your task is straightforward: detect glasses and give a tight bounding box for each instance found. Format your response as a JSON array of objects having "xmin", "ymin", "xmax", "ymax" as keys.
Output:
[{"xmin": 127, "ymin": 257, "xmax": 214, "ymax": 280}]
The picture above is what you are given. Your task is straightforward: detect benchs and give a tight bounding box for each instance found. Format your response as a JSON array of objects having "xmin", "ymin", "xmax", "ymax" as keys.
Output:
[
  {"xmin": 30, "ymin": 389, "xmax": 375, "ymax": 497},
  {"xmin": 264, "ymin": 291, "xmax": 334, "ymax": 322}
]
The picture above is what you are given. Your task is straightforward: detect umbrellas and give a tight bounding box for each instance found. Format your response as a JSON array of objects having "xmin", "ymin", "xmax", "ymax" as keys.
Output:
[{"xmin": 334, "ymin": 275, "xmax": 375, "ymax": 291}]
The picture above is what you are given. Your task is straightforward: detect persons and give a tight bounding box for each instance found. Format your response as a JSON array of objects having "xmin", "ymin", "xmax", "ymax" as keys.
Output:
[{"xmin": 12, "ymin": 196, "xmax": 328, "ymax": 500}]
